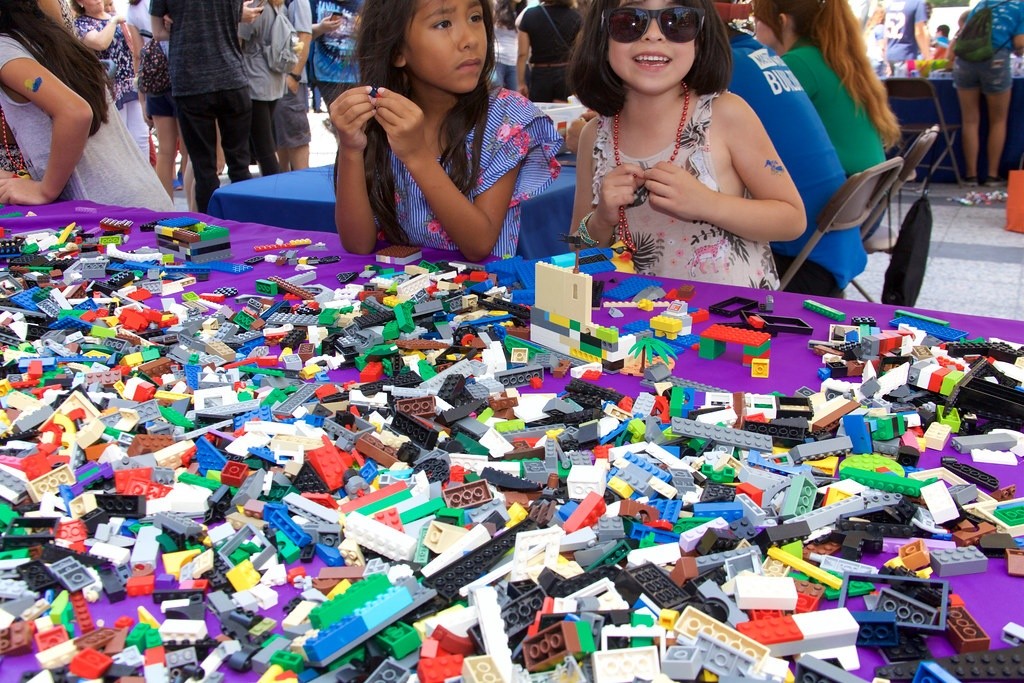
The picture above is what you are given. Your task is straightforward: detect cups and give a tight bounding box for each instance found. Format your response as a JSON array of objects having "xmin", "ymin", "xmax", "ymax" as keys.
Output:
[{"xmin": 906, "ymin": 58, "xmax": 948, "ymax": 78}]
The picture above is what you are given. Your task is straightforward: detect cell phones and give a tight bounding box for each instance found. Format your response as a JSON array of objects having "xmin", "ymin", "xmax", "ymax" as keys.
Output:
[
  {"xmin": 257, "ymin": 0, "xmax": 267, "ymax": 7},
  {"xmin": 329, "ymin": 12, "xmax": 342, "ymax": 21}
]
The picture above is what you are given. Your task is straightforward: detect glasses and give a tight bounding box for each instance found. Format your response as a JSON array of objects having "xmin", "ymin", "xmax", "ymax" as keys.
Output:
[{"xmin": 605, "ymin": 7, "xmax": 705, "ymax": 44}]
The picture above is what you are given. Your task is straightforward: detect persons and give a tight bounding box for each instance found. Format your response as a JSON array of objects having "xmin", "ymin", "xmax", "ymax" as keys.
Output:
[{"xmin": 0, "ymin": 0, "xmax": 1024, "ymax": 298}]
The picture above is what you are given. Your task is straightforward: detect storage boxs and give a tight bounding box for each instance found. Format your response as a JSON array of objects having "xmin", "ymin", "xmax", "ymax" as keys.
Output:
[{"xmin": 531, "ymin": 101, "xmax": 589, "ymax": 141}]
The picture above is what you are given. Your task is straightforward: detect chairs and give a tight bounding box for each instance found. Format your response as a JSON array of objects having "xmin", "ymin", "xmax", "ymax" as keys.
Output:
[
  {"xmin": 776, "ymin": 156, "xmax": 905, "ymax": 298},
  {"xmin": 846, "ymin": 124, "xmax": 942, "ymax": 304},
  {"xmin": 884, "ymin": 78, "xmax": 963, "ymax": 187}
]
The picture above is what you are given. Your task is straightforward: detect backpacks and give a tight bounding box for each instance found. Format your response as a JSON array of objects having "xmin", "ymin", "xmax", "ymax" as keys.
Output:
[
  {"xmin": 881, "ymin": 131, "xmax": 940, "ymax": 307},
  {"xmin": 261, "ymin": 1, "xmax": 304, "ymax": 74},
  {"xmin": 138, "ymin": 38, "xmax": 172, "ymax": 95},
  {"xmin": 953, "ymin": 0, "xmax": 1014, "ymax": 63}
]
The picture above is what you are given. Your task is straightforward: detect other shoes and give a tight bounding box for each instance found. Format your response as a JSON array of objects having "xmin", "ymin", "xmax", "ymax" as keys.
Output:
[
  {"xmin": 961, "ymin": 176, "xmax": 978, "ymax": 186},
  {"xmin": 172, "ymin": 171, "xmax": 184, "ymax": 191},
  {"xmin": 984, "ymin": 175, "xmax": 1007, "ymax": 187}
]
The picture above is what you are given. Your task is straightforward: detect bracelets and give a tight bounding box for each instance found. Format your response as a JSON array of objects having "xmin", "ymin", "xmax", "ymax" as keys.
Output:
[{"xmin": 578, "ymin": 210, "xmax": 615, "ymax": 246}]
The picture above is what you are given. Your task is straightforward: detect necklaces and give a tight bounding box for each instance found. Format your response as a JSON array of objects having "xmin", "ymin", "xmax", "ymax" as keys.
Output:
[
  {"xmin": 1, "ymin": 104, "xmax": 31, "ymax": 180},
  {"xmin": 612, "ymin": 79, "xmax": 691, "ymax": 254}
]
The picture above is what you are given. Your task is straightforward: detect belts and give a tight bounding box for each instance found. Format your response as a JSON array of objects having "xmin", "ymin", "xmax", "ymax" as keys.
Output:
[{"xmin": 528, "ymin": 62, "xmax": 569, "ymax": 67}]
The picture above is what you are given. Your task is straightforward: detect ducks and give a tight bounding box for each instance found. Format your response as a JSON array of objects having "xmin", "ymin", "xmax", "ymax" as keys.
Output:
[
  {"xmin": 609, "ymin": 239, "xmax": 638, "ymax": 274},
  {"xmin": 12, "ymin": 170, "xmax": 31, "ymax": 181}
]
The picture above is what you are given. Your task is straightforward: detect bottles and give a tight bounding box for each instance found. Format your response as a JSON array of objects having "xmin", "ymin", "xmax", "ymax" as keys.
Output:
[{"xmin": 877, "ymin": 60, "xmax": 888, "ymax": 77}]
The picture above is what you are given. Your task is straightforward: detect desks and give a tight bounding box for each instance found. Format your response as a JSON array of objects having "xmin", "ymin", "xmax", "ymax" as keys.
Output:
[
  {"xmin": 0, "ymin": 197, "xmax": 1023, "ymax": 683},
  {"xmin": 889, "ymin": 78, "xmax": 1024, "ymax": 184},
  {"xmin": 207, "ymin": 153, "xmax": 577, "ymax": 260}
]
